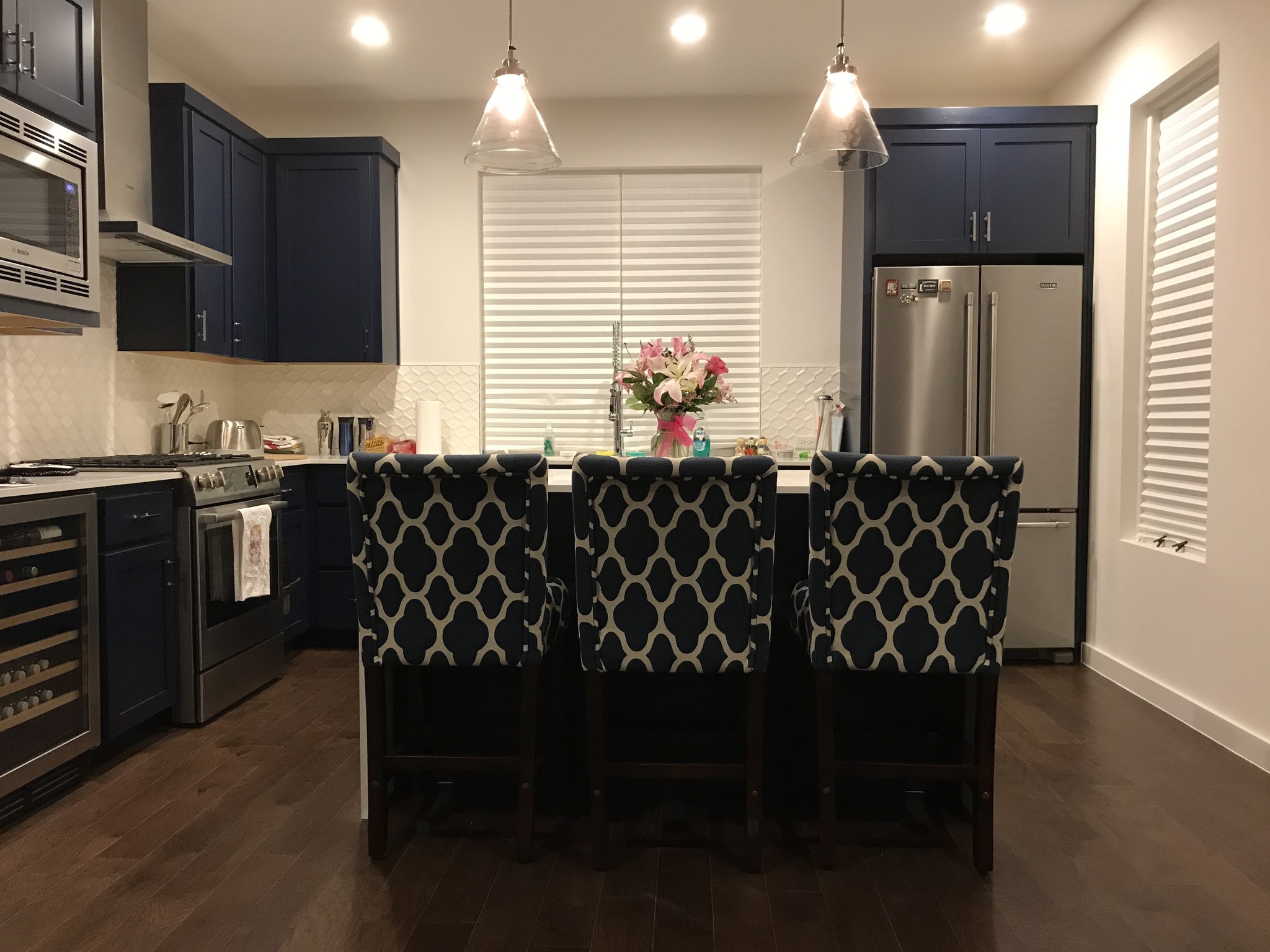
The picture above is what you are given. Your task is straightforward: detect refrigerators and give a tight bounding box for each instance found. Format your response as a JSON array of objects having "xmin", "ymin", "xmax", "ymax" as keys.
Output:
[{"xmin": 870, "ymin": 265, "xmax": 1082, "ymax": 664}]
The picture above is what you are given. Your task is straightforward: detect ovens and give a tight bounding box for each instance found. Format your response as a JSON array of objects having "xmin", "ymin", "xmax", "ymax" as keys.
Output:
[{"xmin": 172, "ymin": 495, "xmax": 286, "ymax": 725}]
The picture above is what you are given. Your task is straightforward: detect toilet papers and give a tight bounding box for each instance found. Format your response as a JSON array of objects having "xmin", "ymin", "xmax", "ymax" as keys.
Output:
[{"xmin": 414, "ymin": 400, "xmax": 444, "ymax": 455}]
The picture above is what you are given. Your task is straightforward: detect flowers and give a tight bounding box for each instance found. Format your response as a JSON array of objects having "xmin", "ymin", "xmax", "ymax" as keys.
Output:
[{"xmin": 615, "ymin": 334, "xmax": 736, "ymax": 412}]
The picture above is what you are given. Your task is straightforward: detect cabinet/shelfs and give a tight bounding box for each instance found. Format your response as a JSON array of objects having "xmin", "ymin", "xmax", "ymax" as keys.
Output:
[
  {"xmin": 116, "ymin": 83, "xmax": 400, "ymax": 363},
  {"xmin": 282, "ymin": 465, "xmax": 361, "ymax": 645},
  {"xmin": 863, "ymin": 105, "xmax": 1098, "ymax": 255},
  {"xmin": 0, "ymin": 0, "xmax": 97, "ymax": 141},
  {"xmin": 100, "ymin": 486, "xmax": 180, "ymax": 737}
]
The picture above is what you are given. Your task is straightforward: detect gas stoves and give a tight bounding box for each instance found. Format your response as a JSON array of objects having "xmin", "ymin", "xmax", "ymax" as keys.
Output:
[{"xmin": 22, "ymin": 451, "xmax": 284, "ymax": 507}]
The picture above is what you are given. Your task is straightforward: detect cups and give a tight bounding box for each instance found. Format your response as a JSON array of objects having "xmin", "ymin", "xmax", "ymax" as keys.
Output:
[{"xmin": 160, "ymin": 424, "xmax": 189, "ymax": 454}]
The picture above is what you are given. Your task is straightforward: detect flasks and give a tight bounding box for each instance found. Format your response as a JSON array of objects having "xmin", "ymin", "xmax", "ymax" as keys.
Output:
[
  {"xmin": 338, "ymin": 417, "xmax": 353, "ymax": 456},
  {"xmin": 358, "ymin": 417, "xmax": 374, "ymax": 451}
]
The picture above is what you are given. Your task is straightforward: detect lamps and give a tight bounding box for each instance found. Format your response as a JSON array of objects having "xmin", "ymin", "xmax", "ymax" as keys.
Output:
[
  {"xmin": 790, "ymin": 0, "xmax": 890, "ymax": 171},
  {"xmin": 463, "ymin": 0, "xmax": 563, "ymax": 175}
]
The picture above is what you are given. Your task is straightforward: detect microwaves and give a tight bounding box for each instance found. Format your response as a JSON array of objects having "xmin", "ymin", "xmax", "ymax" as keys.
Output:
[{"xmin": 0, "ymin": 134, "xmax": 85, "ymax": 281}]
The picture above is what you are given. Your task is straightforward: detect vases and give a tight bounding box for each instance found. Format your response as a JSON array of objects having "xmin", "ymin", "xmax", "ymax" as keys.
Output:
[{"xmin": 651, "ymin": 412, "xmax": 690, "ymax": 457}]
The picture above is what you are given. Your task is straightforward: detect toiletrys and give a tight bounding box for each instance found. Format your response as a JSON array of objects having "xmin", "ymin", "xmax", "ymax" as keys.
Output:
[
  {"xmin": 693, "ymin": 410, "xmax": 712, "ymax": 457},
  {"xmin": 542, "ymin": 422, "xmax": 555, "ymax": 457}
]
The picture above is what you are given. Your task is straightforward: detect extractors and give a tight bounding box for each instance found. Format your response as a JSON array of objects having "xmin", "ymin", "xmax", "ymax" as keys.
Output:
[{"xmin": 93, "ymin": 0, "xmax": 232, "ymax": 268}]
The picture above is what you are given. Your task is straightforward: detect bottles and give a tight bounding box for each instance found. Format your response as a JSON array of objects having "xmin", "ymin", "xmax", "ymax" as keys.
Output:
[
  {"xmin": 317, "ymin": 410, "xmax": 334, "ymax": 456},
  {"xmin": 0, "ymin": 523, "xmax": 70, "ymax": 721},
  {"xmin": 543, "ymin": 423, "xmax": 556, "ymax": 457}
]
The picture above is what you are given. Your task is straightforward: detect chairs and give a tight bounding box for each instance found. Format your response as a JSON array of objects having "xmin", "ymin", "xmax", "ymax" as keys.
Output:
[
  {"xmin": 790, "ymin": 454, "xmax": 1023, "ymax": 877},
  {"xmin": 348, "ymin": 454, "xmax": 567, "ymax": 859},
  {"xmin": 572, "ymin": 449, "xmax": 779, "ymax": 873}
]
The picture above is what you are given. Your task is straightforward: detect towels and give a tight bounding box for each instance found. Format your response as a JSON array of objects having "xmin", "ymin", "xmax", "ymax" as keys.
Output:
[
  {"xmin": 262, "ymin": 434, "xmax": 305, "ymax": 454},
  {"xmin": 228, "ymin": 500, "xmax": 276, "ymax": 604}
]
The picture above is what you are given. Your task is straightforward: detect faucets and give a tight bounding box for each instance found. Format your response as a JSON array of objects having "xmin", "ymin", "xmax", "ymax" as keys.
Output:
[{"xmin": 607, "ymin": 320, "xmax": 635, "ymax": 458}]
